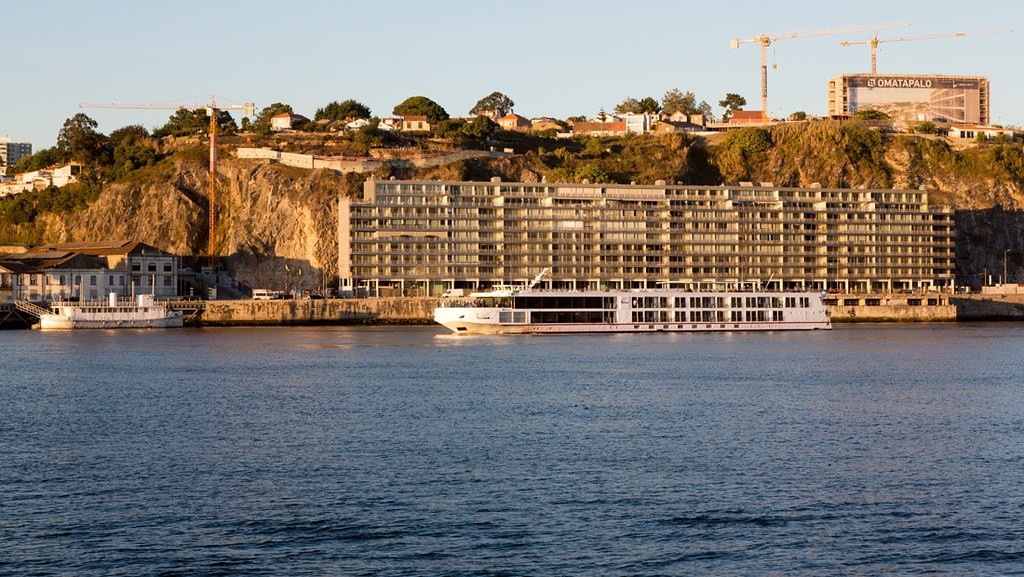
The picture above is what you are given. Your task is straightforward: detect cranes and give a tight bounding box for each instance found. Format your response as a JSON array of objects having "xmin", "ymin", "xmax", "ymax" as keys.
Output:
[
  {"xmin": 78, "ymin": 100, "xmax": 258, "ymax": 272},
  {"xmin": 730, "ymin": 24, "xmax": 917, "ymax": 125},
  {"xmin": 837, "ymin": 33, "xmax": 968, "ymax": 71}
]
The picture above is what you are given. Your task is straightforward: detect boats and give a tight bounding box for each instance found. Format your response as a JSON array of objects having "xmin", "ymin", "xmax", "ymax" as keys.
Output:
[
  {"xmin": 33, "ymin": 272, "xmax": 186, "ymax": 330},
  {"xmin": 433, "ymin": 267, "xmax": 839, "ymax": 333}
]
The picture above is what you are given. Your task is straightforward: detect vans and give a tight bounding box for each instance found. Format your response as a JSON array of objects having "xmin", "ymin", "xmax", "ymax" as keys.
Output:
[{"xmin": 253, "ymin": 289, "xmax": 274, "ymax": 300}]
